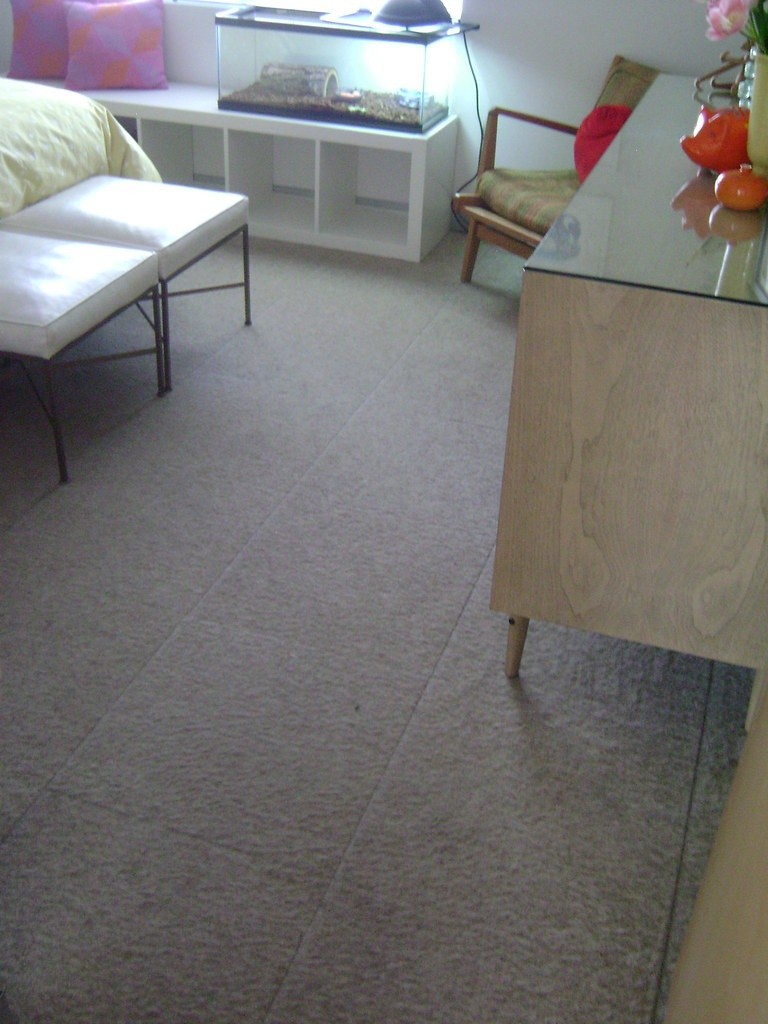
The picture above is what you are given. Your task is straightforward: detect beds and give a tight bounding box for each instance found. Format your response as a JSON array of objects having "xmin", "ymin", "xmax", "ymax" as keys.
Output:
[{"xmin": 0, "ymin": 81, "xmax": 250, "ymax": 482}]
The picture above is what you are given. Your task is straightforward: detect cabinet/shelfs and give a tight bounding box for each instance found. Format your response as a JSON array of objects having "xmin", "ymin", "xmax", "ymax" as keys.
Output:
[
  {"xmin": 488, "ymin": 72, "xmax": 767, "ymax": 736},
  {"xmin": 2, "ymin": 72, "xmax": 457, "ymax": 262}
]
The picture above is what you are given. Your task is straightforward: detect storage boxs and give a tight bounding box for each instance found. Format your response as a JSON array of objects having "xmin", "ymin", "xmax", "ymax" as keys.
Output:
[{"xmin": 216, "ymin": 4, "xmax": 455, "ymax": 134}]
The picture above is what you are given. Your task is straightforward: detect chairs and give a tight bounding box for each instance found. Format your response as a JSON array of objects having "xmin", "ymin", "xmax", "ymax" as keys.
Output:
[{"xmin": 454, "ymin": 55, "xmax": 658, "ymax": 282}]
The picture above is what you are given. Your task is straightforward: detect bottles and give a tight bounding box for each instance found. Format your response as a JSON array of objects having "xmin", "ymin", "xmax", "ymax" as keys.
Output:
[
  {"xmin": 715, "ymin": 163, "xmax": 767, "ymax": 211},
  {"xmin": 709, "ymin": 205, "xmax": 763, "ymax": 248}
]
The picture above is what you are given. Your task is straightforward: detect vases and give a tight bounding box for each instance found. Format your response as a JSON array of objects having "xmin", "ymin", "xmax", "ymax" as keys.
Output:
[{"xmin": 746, "ymin": 54, "xmax": 768, "ymax": 175}]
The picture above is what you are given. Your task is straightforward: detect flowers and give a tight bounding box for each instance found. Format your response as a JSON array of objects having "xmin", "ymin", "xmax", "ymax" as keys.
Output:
[{"xmin": 702, "ymin": 0, "xmax": 768, "ymax": 56}]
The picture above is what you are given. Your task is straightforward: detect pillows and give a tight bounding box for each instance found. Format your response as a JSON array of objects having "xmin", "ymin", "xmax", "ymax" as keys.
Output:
[
  {"xmin": 574, "ymin": 104, "xmax": 632, "ymax": 185},
  {"xmin": 6, "ymin": 0, "xmax": 114, "ymax": 78},
  {"xmin": 60, "ymin": 0, "xmax": 168, "ymax": 89}
]
